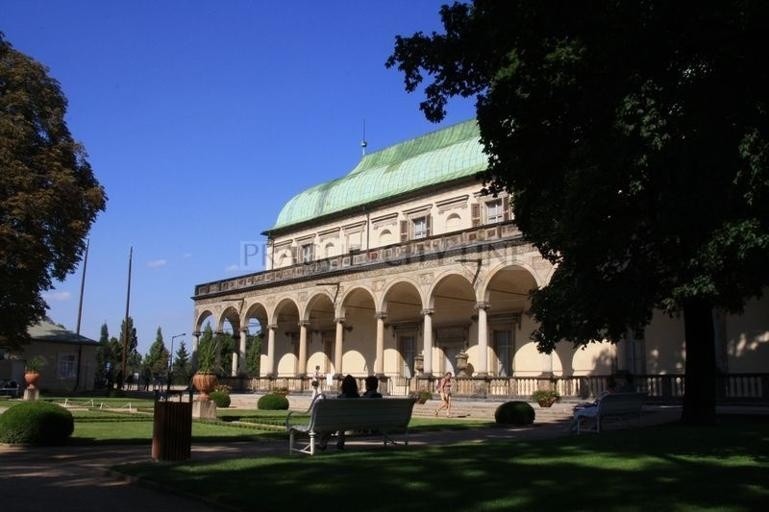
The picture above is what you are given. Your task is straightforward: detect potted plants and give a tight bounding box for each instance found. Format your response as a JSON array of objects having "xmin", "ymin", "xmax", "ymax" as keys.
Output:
[
  {"xmin": 408, "ymin": 389, "xmax": 433, "ymax": 404},
  {"xmin": 529, "ymin": 388, "xmax": 562, "ymax": 408},
  {"xmin": 269, "ymin": 387, "xmax": 289, "ymax": 398},
  {"xmin": 24, "ymin": 355, "xmax": 47, "ymax": 390},
  {"xmin": 192, "ymin": 339, "xmax": 235, "ymax": 400}
]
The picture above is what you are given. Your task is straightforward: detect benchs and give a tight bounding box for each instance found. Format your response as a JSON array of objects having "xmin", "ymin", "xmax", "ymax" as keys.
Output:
[
  {"xmin": 0, "ymin": 384, "xmax": 20, "ymax": 396},
  {"xmin": 573, "ymin": 390, "xmax": 649, "ymax": 434},
  {"xmin": 286, "ymin": 393, "xmax": 418, "ymax": 456}
]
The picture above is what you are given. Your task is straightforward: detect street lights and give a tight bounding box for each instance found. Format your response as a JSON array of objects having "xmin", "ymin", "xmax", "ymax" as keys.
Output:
[{"xmin": 170, "ymin": 333, "xmax": 186, "ymax": 374}]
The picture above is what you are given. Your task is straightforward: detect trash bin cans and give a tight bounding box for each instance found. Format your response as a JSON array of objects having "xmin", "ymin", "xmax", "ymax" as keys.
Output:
[{"xmin": 151, "ymin": 389, "xmax": 193, "ymax": 461}]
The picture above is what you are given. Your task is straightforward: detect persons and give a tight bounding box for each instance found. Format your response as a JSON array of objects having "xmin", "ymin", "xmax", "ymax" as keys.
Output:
[
  {"xmin": 311, "ymin": 366, "xmax": 321, "ymax": 398},
  {"xmin": 360, "ymin": 376, "xmax": 382, "ymax": 398},
  {"xmin": 566, "ymin": 373, "xmax": 635, "ymax": 428},
  {"xmin": 435, "ymin": 372, "xmax": 451, "ymax": 416},
  {"xmin": 143, "ymin": 368, "xmax": 151, "ymax": 391},
  {"xmin": 127, "ymin": 375, "xmax": 133, "ymax": 389},
  {"xmin": 116, "ymin": 370, "xmax": 123, "ymax": 391},
  {"xmin": 315, "ymin": 375, "xmax": 360, "ymax": 450}
]
